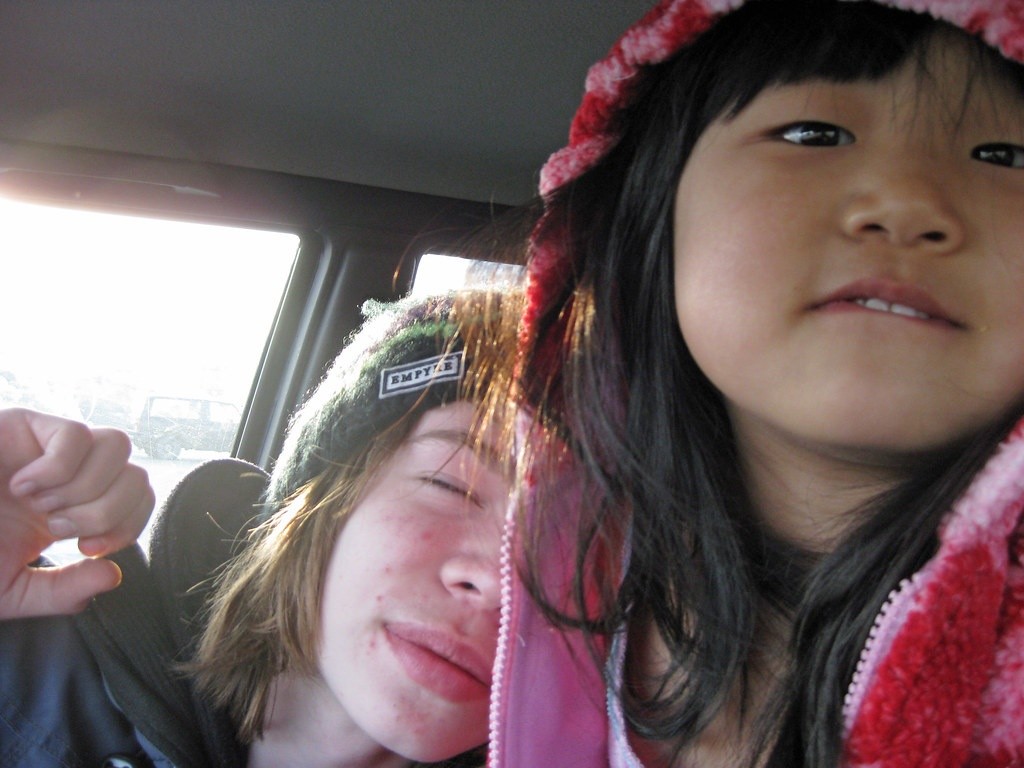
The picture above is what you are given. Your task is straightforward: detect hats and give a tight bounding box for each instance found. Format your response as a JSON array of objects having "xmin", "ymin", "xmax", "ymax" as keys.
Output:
[
  {"xmin": 257, "ymin": 292, "xmax": 525, "ymax": 538},
  {"xmin": 514, "ymin": 2, "xmax": 1021, "ymax": 481}
]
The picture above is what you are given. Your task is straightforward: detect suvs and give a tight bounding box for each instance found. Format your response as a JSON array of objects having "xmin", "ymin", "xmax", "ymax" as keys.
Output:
[{"xmin": 132, "ymin": 396, "xmax": 241, "ymax": 460}]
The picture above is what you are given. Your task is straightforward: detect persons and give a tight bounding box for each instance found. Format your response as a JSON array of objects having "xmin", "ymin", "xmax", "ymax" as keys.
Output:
[
  {"xmin": 488, "ymin": 0, "xmax": 1024, "ymax": 768},
  {"xmin": 2, "ymin": 293, "xmax": 527, "ymax": 768}
]
